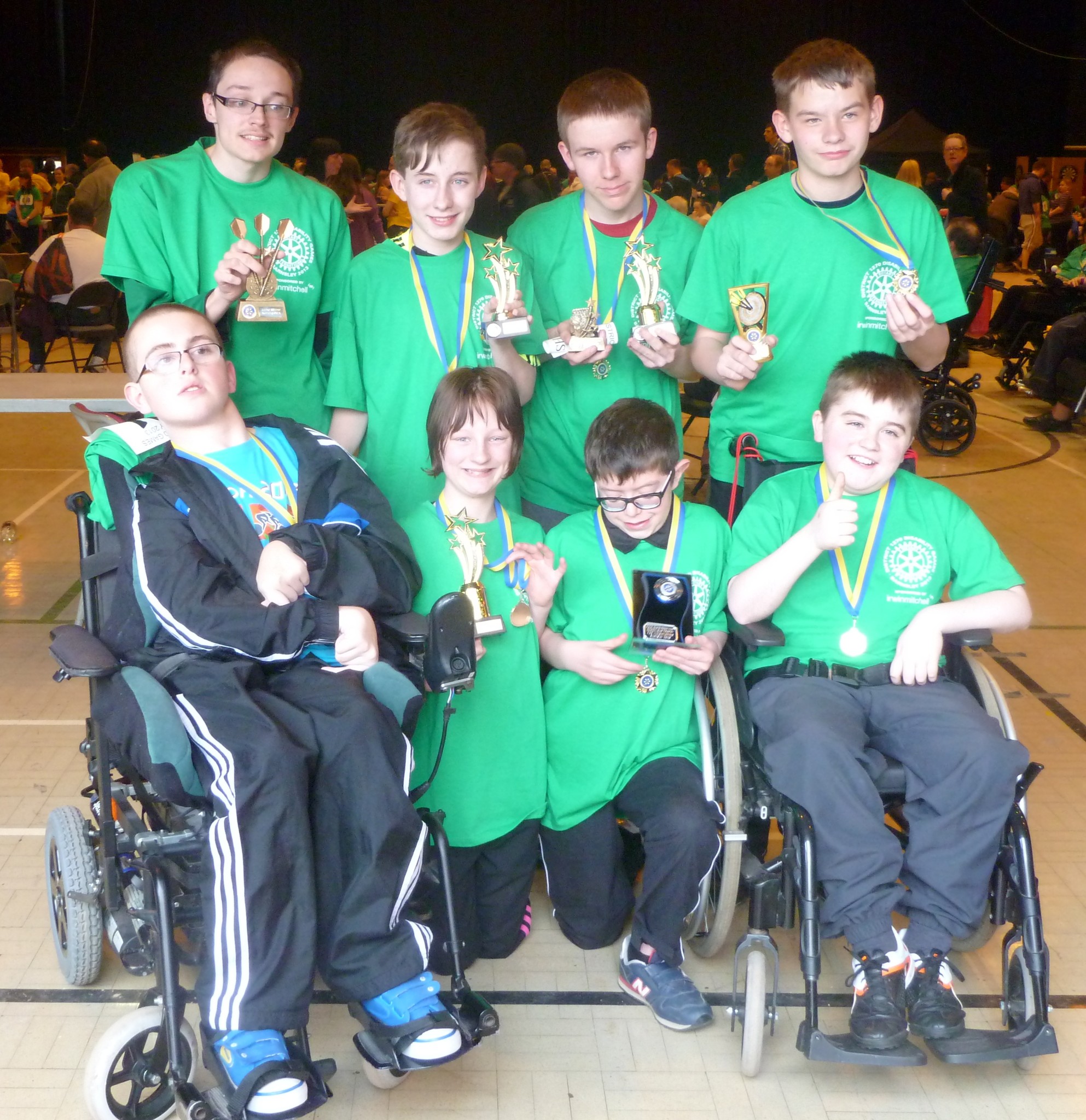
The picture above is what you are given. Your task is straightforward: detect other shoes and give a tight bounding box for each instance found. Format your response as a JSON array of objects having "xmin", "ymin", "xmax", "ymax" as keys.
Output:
[
  {"xmin": 995, "ymin": 265, "xmax": 1012, "ymax": 272},
  {"xmin": 1023, "ymin": 410, "xmax": 1072, "ymax": 432},
  {"xmin": 978, "ymin": 330, "xmax": 1011, "ymax": 345}
]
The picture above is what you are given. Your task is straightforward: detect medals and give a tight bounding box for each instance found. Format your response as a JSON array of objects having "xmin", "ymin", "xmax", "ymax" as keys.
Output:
[
  {"xmin": 634, "ymin": 666, "xmax": 659, "ymax": 693},
  {"xmin": 510, "ymin": 599, "xmax": 533, "ymax": 627},
  {"xmin": 839, "ymin": 627, "xmax": 868, "ymax": 657},
  {"xmin": 893, "ymin": 269, "xmax": 919, "ymax": 295}
]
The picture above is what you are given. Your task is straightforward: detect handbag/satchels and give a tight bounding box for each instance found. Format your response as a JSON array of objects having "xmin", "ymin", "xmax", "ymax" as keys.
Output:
[
  {"xmin": 1049, "ymin": 197, "xmax": 1075, "ymax": 213},
  {"xmin": 20, "ymin": 299, "xmax": 55, "ymax": 343}
]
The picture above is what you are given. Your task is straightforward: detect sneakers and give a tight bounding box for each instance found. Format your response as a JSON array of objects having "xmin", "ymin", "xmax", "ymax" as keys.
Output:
[
  {"xmin": 844, "ymin": 924, "xmax": 915, "ymax": 1050},
  {"xmin": 212, "ymin": 1028, "xmax": 309, "ymax": 1115},
  {"xmin": 898, "ymin": 928, "xmax": 967, "ymax": 1037},
  {"xmin": 1020, "ymin": 268, "xmax": 1037, "ymax": 275},
  {"xmin": 1013, "ymin": 260, "xmax": 1022, "ymax": 271},
  {"xmin": 618, "ymin": 933, "xmax": 715, "ymax": 1033},
  {"xmin": 88, "ymin": 356, "xmax": 112, "ymax": 373},
  {"xmin": 26, "ymin": 364, "xmax": 47, "ymax": 373},
  {"xmin": 364, "ymin": 968, "xmax": 463, "ymax": 1061}
]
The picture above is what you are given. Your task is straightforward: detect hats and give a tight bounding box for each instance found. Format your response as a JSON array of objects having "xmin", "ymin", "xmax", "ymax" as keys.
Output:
[
  {"xmin": 496, "ymin": 143, "xmax": 525, "ymax": 170},
  {"xmin": 667, "ymin": 196, "xmax": 688, "ymax": 216}
]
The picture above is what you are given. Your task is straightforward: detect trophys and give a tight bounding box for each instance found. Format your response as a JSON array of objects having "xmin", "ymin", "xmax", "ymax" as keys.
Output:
[
  {"xmin": 444, "ymin": 508, "xmax": 506, "ymax": 638},
  {"xmin": 623, "ymin": 234, "xmax": 678, "ymax": 346},
  {"xmin": 229, "ymin": 214, "xmax": 294, "ymax": 322},
  {"xmin": 728, "ymin": 282, "xmax": 774, "ymax": 363},
  {"xmin": 481, "ymin": 235, "xmax": 532, "ymax": 340},
  {"xmin": 568, "ymin": 297, "xmax": 606, "ymax": 352}
]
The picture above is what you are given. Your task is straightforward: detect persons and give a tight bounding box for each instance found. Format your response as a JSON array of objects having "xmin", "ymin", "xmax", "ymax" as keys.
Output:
[
  {"xmin": 540, "ymin": 397, "xmax": 736, "ymax": 1031},
  {"xmin": 676, "ymin": 36, "xmax": 971, "ymax": 524},
  {"xmin": 327, "ymin": 102, "xmax": 549, "ymax": 527},
  {"xmin": 1, "ymin": 120, "xmax": 1086, "ymax": 433},
  {"xmin": 505, "ymin": 70, "xmax": 704, "ymax": 533},
  {"xmin": 102, "ymin": 41, "xmax": 355, "ymax": 433},
  {"xmin": 122, "ymin": 302, "xmax": 462, "ymax": 1114},
  {"xmin": 728, "ymin": 353, "xmax": 1034, "ymax": 1052},
  {"xmin": 398, "ymin": 363, "xmax": 566, "ymax": 975}
]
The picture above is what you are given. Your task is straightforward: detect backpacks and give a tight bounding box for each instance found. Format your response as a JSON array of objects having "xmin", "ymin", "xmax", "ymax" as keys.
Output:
[{"xmin": 33, "ymin": 233, "xmax": 73, "ymax": 302}]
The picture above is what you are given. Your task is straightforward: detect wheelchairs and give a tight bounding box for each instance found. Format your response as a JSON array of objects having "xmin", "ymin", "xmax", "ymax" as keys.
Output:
[
  {"xmin": 994, "ymin": 325, "xmax": 1086, "ymax": 431},
  {"xmin": 911, "ymin": 249, "xmax": 1001, "ymax": 455},
  {"xmin": 682, "ymin": 605, "xmax": 1060, "ymax": 1077},
  {"xmin": 47, "ymin": 496, "xmax": 502, "ymax": 1118}
]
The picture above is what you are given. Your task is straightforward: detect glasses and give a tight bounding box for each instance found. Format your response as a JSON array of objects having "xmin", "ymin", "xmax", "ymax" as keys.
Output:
[
  {"xmin": 594, "ymin": 469, "xmax": 672, "ymax": 513},
  {"xmin": 137, "ymin": 343, "xmax": 223, "ymax": 383},
  {"xmin": 208, "ymin": 90, "xmax": 295, "ymax": 120},
  {"xmin": 490, "ymin": 159, "xmax": 506, "ymax": 167},
  {"xmin": 943, "ymin": 146, "xmax": 964, "ymax": 153},
  {"xmin": 763, "ymin": 163, "xmax": 777, "ymax": 168}
]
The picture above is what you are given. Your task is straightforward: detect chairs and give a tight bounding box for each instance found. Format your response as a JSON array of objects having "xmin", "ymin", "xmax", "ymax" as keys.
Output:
[
  {"xmin": 38, "ymin": 279, "xmax": 127, "ymax": 373},
  {"xmin": 0, "ymin": 253, "xmax": 30, "ymax": 273},
  {"xmin": 0, "ymin": 278, "xmax": 20, "ymax": 373},
  {"xmin": 680, "ymin": 393, "xmax": 712, "ymax": 495}
]
